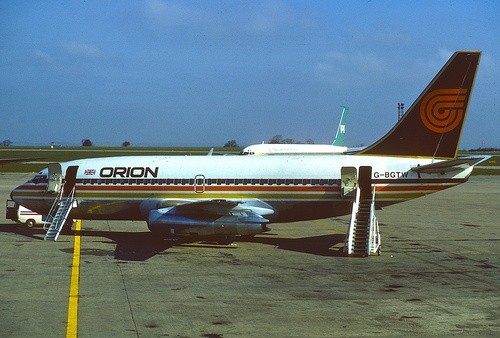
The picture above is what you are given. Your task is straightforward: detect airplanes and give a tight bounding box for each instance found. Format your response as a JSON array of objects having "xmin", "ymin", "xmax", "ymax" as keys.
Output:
[{"xmin": 11, "ymin": 51, "xmax": 495, "ymax": 249}]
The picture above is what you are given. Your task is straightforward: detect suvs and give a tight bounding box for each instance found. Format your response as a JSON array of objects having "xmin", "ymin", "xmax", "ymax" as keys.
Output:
[{"xmin": 4, "ymin": 199, "xmax": 73, "ymax": 228}]
